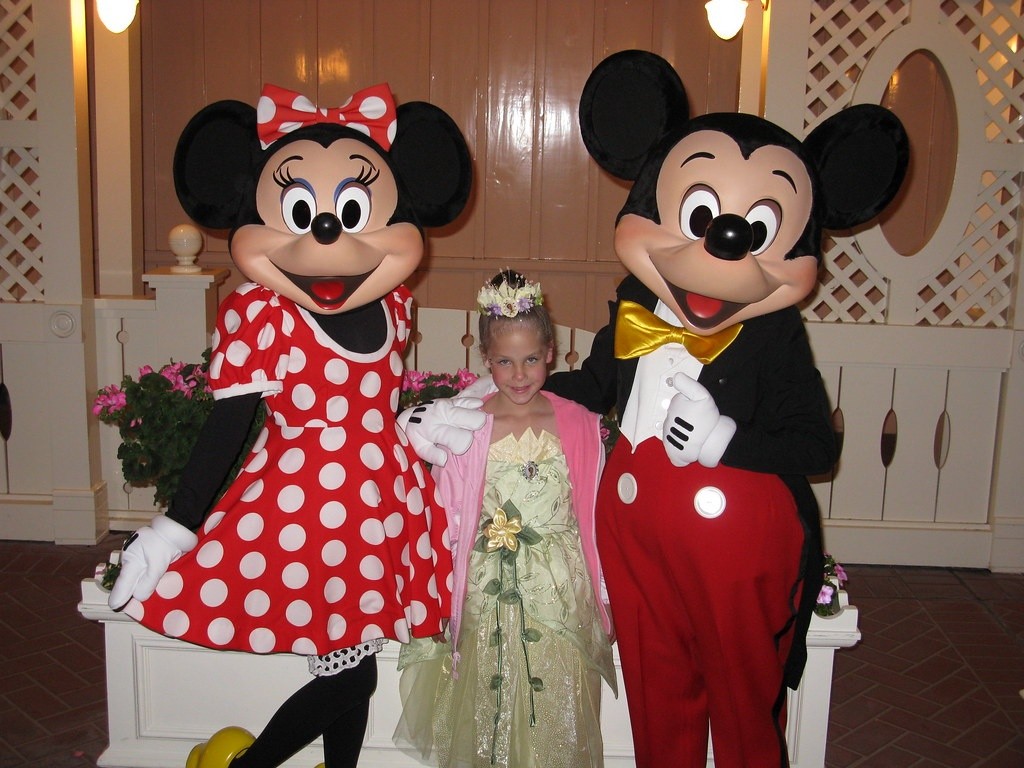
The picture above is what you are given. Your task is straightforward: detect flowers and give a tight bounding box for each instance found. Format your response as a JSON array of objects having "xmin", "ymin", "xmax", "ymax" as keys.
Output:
[
  {"xmin": 476, "ymin": 277, "xmax": 543, "ymax": 317},
  {"xmin": 90, "ymin": 362, "xmax": 848, "ymax": 616}
]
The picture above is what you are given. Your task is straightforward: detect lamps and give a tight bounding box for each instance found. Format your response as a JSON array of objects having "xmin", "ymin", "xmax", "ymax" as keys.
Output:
[
  {"xmin": 97, "ymin": 0, "xmax": 139, "ymax": 33},
  {"xmin": 704, "ymin": 0, "xmax": 769, "ymax": 40}
]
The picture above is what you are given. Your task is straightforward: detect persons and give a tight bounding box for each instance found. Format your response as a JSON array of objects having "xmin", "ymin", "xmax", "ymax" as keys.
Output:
[{"xmin": 391, "ymin": 267, "xmax": 617, "ymax": 768}]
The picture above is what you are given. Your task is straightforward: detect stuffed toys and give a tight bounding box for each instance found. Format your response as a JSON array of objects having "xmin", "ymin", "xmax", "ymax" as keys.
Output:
[
  {"xmin": 455, "ymin": 49, "xmax": 913, "ymax": 768},
  {"xmin": 107, "ymin": 82, "xmax": 486, "ymax": 768}
]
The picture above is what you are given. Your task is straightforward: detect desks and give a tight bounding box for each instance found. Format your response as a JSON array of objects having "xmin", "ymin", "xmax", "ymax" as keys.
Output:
[{"xmin": 77, "ymin": 569, "xmax": 860, "ymax": 768}]
